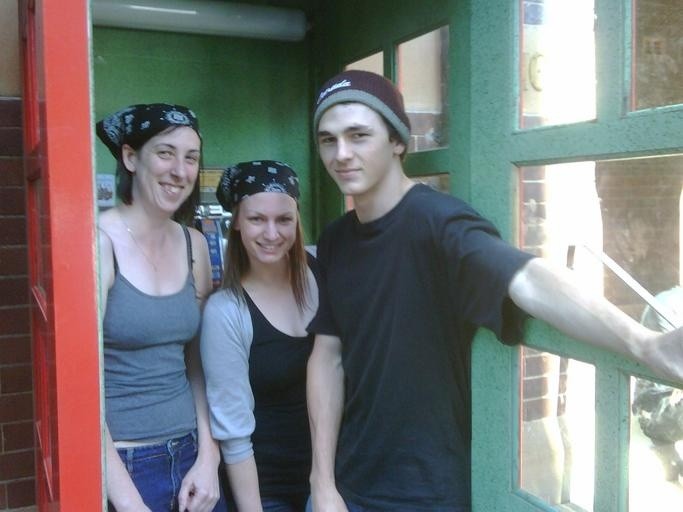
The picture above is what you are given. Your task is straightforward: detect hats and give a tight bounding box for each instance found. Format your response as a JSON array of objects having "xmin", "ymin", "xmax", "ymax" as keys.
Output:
[{"xmin": 312, "ymin": 70, "xmax": 411, "ymax": 152}]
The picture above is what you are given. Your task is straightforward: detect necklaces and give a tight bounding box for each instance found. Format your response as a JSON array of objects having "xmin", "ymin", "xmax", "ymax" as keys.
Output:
[{"xmin": 121, "ymin": 214, "xmax": 158, "ymax": 274}]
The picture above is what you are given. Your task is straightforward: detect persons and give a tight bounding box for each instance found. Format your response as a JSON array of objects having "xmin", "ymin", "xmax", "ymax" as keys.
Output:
[
  {"xmin": 305, "ymin": 70, "xmax": 683, "ymax": 512},
  {"xmin": 95, "ymin": 104, "xmax": 230, "ymax": 512},
  {"xmin": 199, "ymin": 160, "xmax": 318, "ymax": 512}
]
